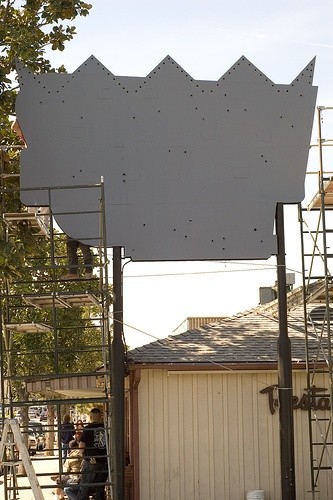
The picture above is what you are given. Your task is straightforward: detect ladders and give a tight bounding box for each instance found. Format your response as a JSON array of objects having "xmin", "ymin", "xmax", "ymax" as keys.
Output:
[{"xmin": 1, "ymin": 417, "xmax": 47, "ymax": 500}]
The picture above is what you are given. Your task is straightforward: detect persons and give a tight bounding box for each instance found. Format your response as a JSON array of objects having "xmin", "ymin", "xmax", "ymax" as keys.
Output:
[
  {"xmin": 70, "ymin": 408, "xmax": 111, "ymax": 500},
  {"xmin": 61, "ymin": 235, "xmax": 94, "ymax": 279},
  {"xmin": 51, "ymin": 415, "xmax": 85, "ymax": 500}
]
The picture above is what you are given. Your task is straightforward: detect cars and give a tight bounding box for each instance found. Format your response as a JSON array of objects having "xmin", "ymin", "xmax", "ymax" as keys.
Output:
[{"xmin": 0, "ymin": 404, "xmax": 47, "ymax": 456}]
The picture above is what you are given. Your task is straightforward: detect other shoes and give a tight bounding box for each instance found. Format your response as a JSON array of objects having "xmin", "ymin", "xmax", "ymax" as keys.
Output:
[{"xmin": 50, "ymin": 476, "xmax": 58, "ymax": 481}]
